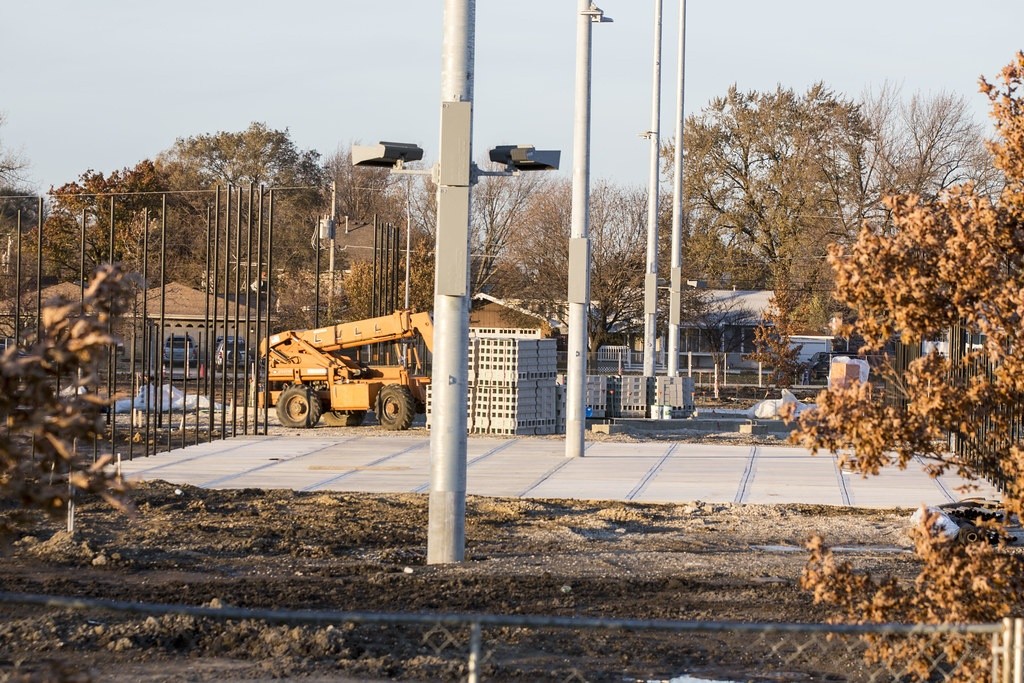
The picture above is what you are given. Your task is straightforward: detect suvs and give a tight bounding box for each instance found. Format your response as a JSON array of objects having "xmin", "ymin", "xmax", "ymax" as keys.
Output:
[
  {"xmin": 164, "ymin": 335, "xmax": 199, "ymax": 367},
  {"xmin": 215, "ymin": 339, "xmax": 249, "ymax": 373}
]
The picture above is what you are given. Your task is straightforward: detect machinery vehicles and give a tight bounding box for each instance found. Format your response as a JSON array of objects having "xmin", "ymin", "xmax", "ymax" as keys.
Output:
[{"xmin": 250, "ymin": 310, "xmax": 436, "ymax": 430}]
